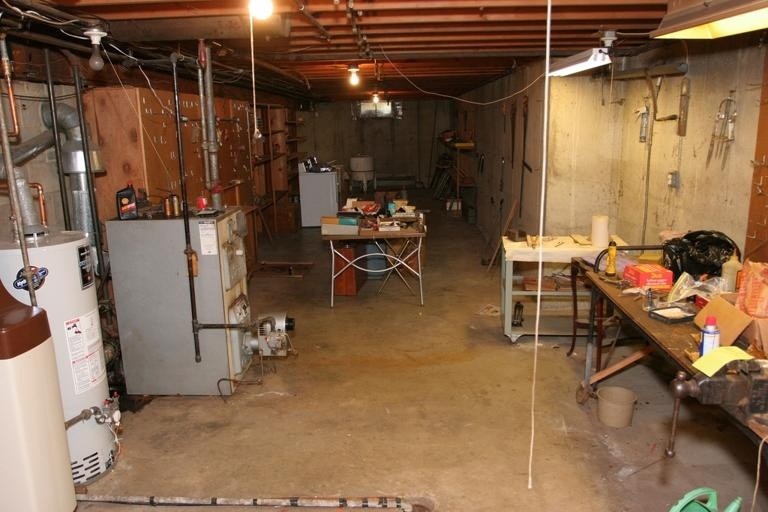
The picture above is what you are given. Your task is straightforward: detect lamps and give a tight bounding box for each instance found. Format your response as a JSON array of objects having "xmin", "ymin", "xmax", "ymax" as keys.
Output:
[
  {"xmin": 601, "ymin": 31, "xmax": 617, "ymax": 54},
  {"xmin": 546, "ymin": 47, "xmax": 612, "ymax": 77},
  {"xmin": 372, "ymin": 92, "xmax": 379, "ymax": 103},
  {"xmin": 347, "ymin": 64, "xmax": 361, "ymax": 87},
  {"xmin": 84, "ymin": 29, "xmax": 107, "ymax": 70},
  {"xmin": 651, "ymin": 1, "xmax": 768, "ymax": 40}
]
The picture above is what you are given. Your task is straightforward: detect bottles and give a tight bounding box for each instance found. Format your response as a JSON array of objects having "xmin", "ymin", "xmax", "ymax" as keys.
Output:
[
  {"xmin": 720, "ymin": 246, "xmax": 744, "ymax": 292},
  {"xmin": 164, "ymin": 194, "xmax": 180, "ymax": 218},
  {"xmin": 699, "ymin": 316, "xmax": 721, "ymax": 358}
]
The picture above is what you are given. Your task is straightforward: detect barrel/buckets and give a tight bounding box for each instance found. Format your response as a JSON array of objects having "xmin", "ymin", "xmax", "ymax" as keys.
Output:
[
  {"xmin": 351, "ymin": 156, "xmax": 374, "ymax": 181},
  {"xmin": 598, "ymin": 386, "xmax": 637, "ymax": 428}
]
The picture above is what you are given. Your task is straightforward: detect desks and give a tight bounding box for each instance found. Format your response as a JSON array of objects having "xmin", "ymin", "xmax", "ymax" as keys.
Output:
[
  {"xmin": 323, "ymin": 217, "xmax": 426, "ymax": 308},
  {"xmin": 570, "ymin": 253, "xmax": 661, "ymax": 374},
  {"xmin": 503, "ymin": 231, "xmax": 630, "ymax": 342}
]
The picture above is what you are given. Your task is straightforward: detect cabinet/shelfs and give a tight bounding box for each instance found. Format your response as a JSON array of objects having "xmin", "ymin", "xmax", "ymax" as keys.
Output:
[
  {"xmin": 435, "ymin": 134, "xmax": 478, "ymax": 220},
  {"xmin": 83, "ymin": 84, "xmax": 302, "ymax": 262}
]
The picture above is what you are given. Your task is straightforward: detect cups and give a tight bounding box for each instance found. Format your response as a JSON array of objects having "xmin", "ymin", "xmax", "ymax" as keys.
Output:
[{"xmin": 641, "ymin": 287, "xmax": 660, "ymax": 313}]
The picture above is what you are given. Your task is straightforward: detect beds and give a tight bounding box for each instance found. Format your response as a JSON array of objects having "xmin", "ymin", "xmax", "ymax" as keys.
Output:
[{"xmin": 585, "ymin": 242, "xmax": 768, "ymax": 459}]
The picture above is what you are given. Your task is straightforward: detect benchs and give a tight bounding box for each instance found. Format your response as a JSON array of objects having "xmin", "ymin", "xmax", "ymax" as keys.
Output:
[{"xmin": 363, "ymin": 176, "xmax": 415, "ymax": 190}]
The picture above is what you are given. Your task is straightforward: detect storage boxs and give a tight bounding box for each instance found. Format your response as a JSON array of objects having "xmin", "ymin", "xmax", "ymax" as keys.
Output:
[
  {"xmin": 319, "ymin": 215, "xmax": 359, "ymax": 235},
  {"xmin": 695, "ymin": 290, "xmax": 767, "ymax": 354},
  {"xmin": 624, "ymin": 264, "xmax": 673, "ymax": 290}
]
